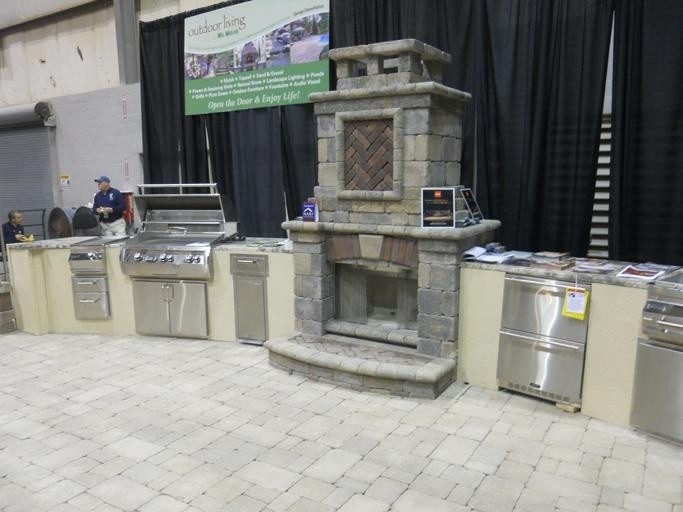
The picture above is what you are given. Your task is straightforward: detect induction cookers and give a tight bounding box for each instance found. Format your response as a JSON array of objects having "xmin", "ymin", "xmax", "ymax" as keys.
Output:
[{"xmin": 119, "ymin": 235, "xmax": 214, "ymax": 264}]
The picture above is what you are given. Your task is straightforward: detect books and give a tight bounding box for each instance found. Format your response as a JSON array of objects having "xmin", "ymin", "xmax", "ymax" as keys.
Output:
[{"xmin": 462, "ymin": 245, "xmax": 683, "ymax": 290}]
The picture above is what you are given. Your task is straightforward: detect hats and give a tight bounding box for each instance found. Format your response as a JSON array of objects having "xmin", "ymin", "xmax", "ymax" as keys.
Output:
[{"xmin": 94, "ymin": 176, "xmax": 110, "ymax": 183}]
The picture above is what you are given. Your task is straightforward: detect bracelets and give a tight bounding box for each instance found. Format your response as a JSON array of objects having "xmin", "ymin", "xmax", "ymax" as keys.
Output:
[{"xmin": 110, "ymin": 208, "xmax": 113, "ymax": 214}]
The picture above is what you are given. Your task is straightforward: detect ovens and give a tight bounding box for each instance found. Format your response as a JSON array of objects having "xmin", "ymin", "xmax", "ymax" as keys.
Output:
[
  {"xmin": 629, "ymin": 339, "xmax": 683, "ymax": 444},
  {"xmin": 496, "ymin": 274, "xmax": 591, "ymax": 407}
]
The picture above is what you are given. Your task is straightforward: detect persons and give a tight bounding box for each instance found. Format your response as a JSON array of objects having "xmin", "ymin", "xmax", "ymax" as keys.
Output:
[
  {"xmin": 93, "ymin": 176, "xmax": 126, "ymax": 236},
  {"xmin": 2, "ymin": 210, "xmax": 28, "ymax": 244}
]
